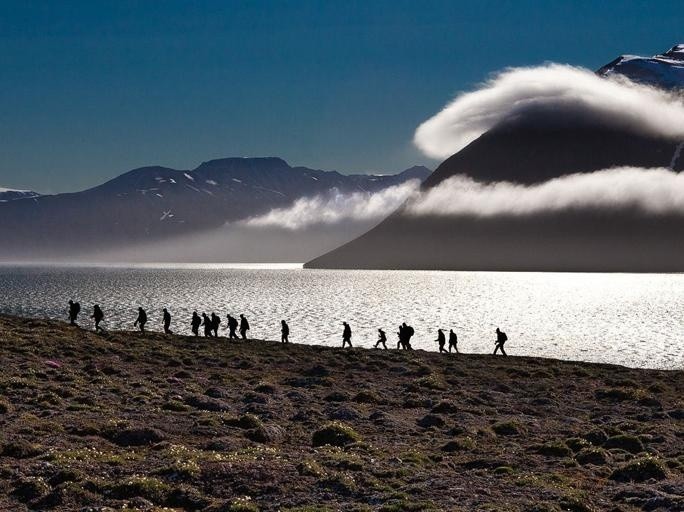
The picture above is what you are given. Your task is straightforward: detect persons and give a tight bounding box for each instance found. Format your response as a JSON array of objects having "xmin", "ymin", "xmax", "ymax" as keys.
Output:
[
  {"xmin": 374, "ymin": 323, "xmax": 413, "ymax": 350},
  {"xmin": 281, "ymin": 320, "xmax": 289, "ymax": 343},
  {"xmin": 134, "ymin": 307, "xmax": 249, "ymax": 339},
  {"xmin": 68, "ymin": 300, "xmax": 77, "ymax": 324},
  {"xmin": 435, "ymin": 329, "xmax": 448, "ymax": 353},
  {"xmin": 90, "ymin": 305, "xmax": 103, "ymax": 332},
  {"xmin": 449, "ymin": 330, "xmax": 459, "ymax": 353},
  {"xmin": 493, "ymin": 328, "xmax": 507, "ymax": 355},
  {"xmin": 342, "ymin": 322, "xmax": 352, "ymax": 348}
]
